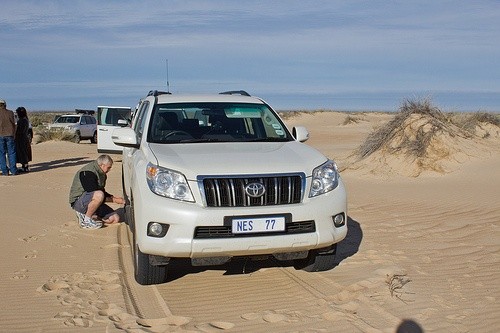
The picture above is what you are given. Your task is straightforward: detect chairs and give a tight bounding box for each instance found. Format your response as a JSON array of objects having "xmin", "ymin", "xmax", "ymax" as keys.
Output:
[
  {"xmin": 161, "ymin": 117, "xmax": 201, "ymax": 141},
  {"xmin": 213, "ymin": 119, "xmax": 247, "ymax": 136}
]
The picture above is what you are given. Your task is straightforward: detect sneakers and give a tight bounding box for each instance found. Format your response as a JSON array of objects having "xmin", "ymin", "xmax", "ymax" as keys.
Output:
[
  {"xmin": 80, "ymin": 218, "xmax": 102, "ymax": 230},
  {"xmin": 76, "ymin": 212, "xmax": 85, "ymax": 224}
]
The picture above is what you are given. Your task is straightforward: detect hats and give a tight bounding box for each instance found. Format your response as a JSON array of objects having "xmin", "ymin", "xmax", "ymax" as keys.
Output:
[{"xmin": 0, "ymin": 100, "xmax": 5, "ymax": 104}]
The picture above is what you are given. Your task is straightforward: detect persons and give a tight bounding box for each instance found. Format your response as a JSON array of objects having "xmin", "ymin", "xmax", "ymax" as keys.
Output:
[
  {"xmin": 14, "ymin": 106, "xmax": 32, "ymax": 172},
  {"xmin": 0, "ymin": 100, "xmax": 22, "ymax": 176},
  {"xmin": 69, "ymin": 155, "xmax": 126, "ymax": 230}
]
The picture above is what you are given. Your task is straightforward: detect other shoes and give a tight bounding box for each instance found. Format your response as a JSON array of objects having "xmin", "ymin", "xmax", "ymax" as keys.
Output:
[
  {"xmin": 2, "ymin": 172, "xmax": 8, "ymax": 176},
  {"xmin": 13, "ymin": 169, "xmax": 22, "ymax": 175}
]
